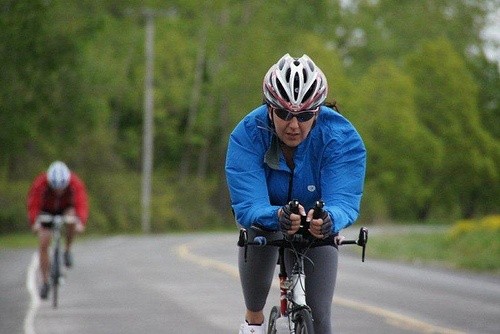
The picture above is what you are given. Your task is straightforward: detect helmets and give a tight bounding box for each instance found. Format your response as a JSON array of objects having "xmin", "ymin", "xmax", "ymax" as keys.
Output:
[
  {"xmin": 263, "ymin": 53, "xmax": 328, "ymax": 114},
  {"xmin": 47, "ymin": 161, "xmax": 70, "ymax": 189}
]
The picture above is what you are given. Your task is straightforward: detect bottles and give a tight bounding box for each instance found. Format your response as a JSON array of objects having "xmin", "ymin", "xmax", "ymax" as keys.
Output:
[{"xmin": 275, "ymin": 317, "xmax": 295, "ymax": 334}]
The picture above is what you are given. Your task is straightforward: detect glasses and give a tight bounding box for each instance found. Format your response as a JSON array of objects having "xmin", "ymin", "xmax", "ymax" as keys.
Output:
[{"xmin": 268, "ymin": 106, "xmax": 317, "ymax": 122}]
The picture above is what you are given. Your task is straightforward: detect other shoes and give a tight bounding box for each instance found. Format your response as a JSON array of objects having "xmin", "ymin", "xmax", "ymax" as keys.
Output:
[
  {"xmin": 63, "ymin": 252, "xmax": 72, "ymax": 268},
  {"xmin": 239, "ymin": 314, "xmax": 266, "ymax": 334},
  {"xmin": 40, "ymin": 283, "xmax": 50, "ymax": 298}
]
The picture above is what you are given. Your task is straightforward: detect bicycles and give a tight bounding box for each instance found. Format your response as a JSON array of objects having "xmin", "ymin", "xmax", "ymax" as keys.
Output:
[
  {"xmin": 36, "ymin": 214, "xmax": 78, "ymax": 309},
  {"xmin": 237, "ymin": 198, "xmax": 367, "ymax": 334}
]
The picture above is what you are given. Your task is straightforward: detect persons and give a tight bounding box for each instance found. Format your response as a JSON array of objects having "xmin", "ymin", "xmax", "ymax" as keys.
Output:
[
  {"xmin": 28, "ymin": 162, "xmax": 89, "ymax": 298},
  {"xmin": 225, "ymin": 53, "xmax": 367, "ymax": 334}
]
